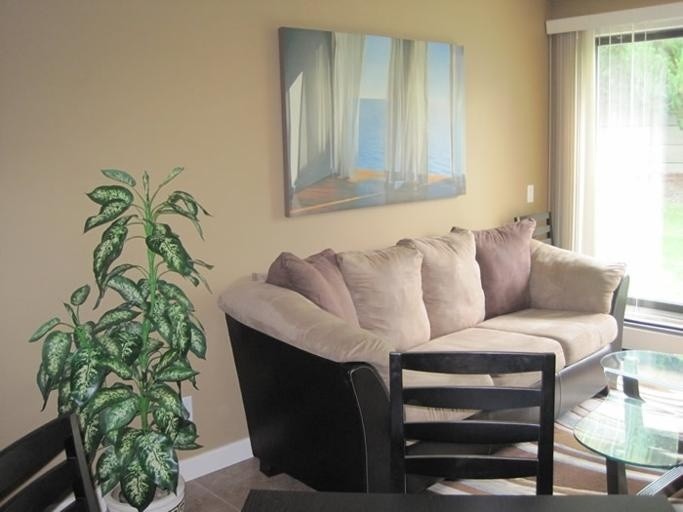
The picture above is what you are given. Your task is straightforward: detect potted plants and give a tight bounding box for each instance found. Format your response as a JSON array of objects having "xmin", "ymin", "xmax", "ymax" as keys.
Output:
[{"xmin": 30, "ymin": 165, "xmax": 217, "ymax": 510}]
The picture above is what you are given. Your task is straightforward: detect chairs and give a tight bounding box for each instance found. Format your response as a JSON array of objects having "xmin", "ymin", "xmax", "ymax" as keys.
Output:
[
  {"xmin": 1, "ymin": 408, "xmax": 102, "ymax": 511},
  {"xmin": 389, "ymin": 351, "xmax": 557, "ymax": 494}
]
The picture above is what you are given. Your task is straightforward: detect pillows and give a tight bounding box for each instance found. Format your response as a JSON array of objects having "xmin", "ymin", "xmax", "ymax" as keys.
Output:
[{"xmin": 265, "ymin": 215, "xmax": 538, "ymax": 354}]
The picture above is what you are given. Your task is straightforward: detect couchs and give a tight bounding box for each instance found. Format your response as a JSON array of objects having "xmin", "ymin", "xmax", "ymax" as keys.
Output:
[{"xmin": 219, "ymin": 238, "xmax": 632, "ymax": 495}]
